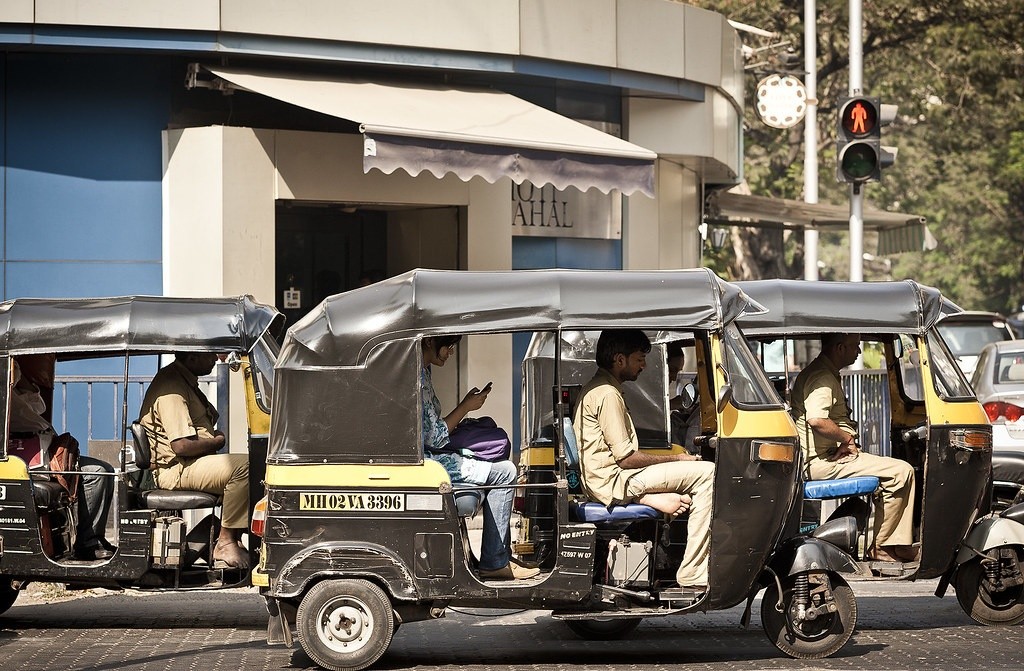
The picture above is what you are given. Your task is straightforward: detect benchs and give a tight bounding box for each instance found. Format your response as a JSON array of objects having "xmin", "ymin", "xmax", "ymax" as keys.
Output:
[
  {"xmin": 418, "ymin": 453, "xmax": 493, "ymax": 516},
  {"xmin": 804, "ymin": 476, "xmax": 879, "ymax": 500},
  {"xmin": 8, "ymin": 454, "xmax": 80, "ymax": 511}
]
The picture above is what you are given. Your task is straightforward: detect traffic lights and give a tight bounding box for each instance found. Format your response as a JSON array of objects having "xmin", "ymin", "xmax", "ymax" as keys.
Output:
[
  {"xmin": 880, "ymin": 104, "xmax": 900, "ymax": 170},
  {"xmin": 835, "ymin": 96, "xmax": 881, "ymax": 183}
]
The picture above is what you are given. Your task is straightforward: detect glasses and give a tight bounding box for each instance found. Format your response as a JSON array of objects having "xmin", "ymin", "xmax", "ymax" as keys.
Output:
[{"xmin": 448, "ymin": 344, "xmax": 456, "ymax": 350}]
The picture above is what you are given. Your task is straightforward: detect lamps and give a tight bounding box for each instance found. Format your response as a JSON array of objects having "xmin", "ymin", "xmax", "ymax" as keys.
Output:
[{"xmin": 703, "ymin": 228, "xmax": 730, "ymax": 255}]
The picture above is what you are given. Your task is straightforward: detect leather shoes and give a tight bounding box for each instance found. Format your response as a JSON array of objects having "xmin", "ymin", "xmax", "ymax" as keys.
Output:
[
  {"xmin": 73, "ymin": 541, "xmax": 113, "ymax": 560},
  {"xmin": 480, "ymin": 556, "xmax": 541, "ymax": 579}
]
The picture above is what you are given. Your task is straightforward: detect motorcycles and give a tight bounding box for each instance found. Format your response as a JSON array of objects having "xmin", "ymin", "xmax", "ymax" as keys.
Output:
[
  {"xmin": 250, "ymin": 267, "xmax": 864, "ymax": 671},
  {"xmin": 0, "ymin": 294, "xmax": 289, "ymax": 615},
  {"xmin": 505, "ymin": 279, "xmax": 1024, "ymax": 626}
]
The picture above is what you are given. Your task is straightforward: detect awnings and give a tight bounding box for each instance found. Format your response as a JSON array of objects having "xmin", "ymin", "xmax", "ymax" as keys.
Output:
[
  {"xmin": 203, "ymin": 65, "xmax": 658, "ymax": 199},
  {"xmin": 716, "ymin": 192, "xmax": 926, "ymax": 256}
]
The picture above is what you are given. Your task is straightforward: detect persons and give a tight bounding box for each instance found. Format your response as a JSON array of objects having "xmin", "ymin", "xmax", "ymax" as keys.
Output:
[
  {"xmin": 792, "ymin": 333, "xmax": 919, "ymax": 561},
  {"xmin": 573, "ymin": 329, "xmax": 715, "ymax": 587},
  {"xmin": 666, "ymin": 345, "xmax": 685, "ymax": 387},
  {"xmin": 420, "ymin": 335, "xmax": 541, "ymax": 578},
  {"xmin": 9, "ymin": 380, "xmax": 117, "ymax": 559},
  {"xmin": 139, "ymin": 352, "xmax": 250, "ymax": 567}
]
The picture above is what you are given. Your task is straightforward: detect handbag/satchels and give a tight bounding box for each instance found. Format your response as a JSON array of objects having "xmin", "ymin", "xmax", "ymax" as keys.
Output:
[
  {"xmin": 49, "ymin": 431, "xmax": 79, "ymax": 478},
  {"xmin": 6, "ymin": 435, "xmax": 51, "ymax": 480},
  {"xmin": 445, "ymin": 416, "xmax": 511, "ymax": 464}
]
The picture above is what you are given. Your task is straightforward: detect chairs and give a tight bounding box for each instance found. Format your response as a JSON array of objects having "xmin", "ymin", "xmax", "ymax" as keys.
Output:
[
  {"xmin": 1009, "ymin": 363, "xmax": 1024, "ymax": 381},
  {"xmin": 128, "ymin": 420, "xmax": 224, "ymax": 512},
  {"xmin": 555, "ymin": 418, "xmax": 659, "ymax": 520}
]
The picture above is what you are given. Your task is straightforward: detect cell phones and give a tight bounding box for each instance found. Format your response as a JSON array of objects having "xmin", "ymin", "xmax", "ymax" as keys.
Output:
[{"xmin": 481, "ymin": 382, "xmax": 493, "ymax": 392}]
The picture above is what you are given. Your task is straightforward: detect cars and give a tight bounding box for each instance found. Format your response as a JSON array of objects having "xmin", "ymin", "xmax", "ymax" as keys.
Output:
[{"xmin": 934, "ymin": 311, "xmax": 1024, "ymax": 483}]
